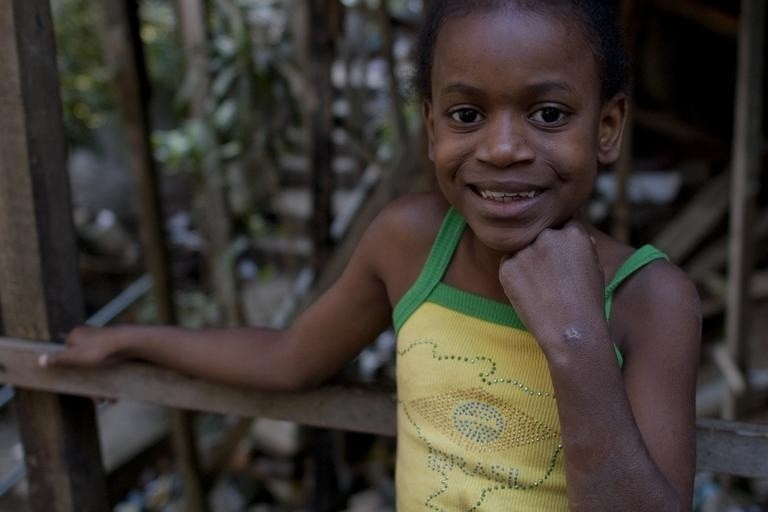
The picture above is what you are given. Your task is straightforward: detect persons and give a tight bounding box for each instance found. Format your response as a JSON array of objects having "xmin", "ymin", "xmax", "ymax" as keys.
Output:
[{"xmin": 37, "ymin": 0, "xmax": 703, "ymax": 512}]
